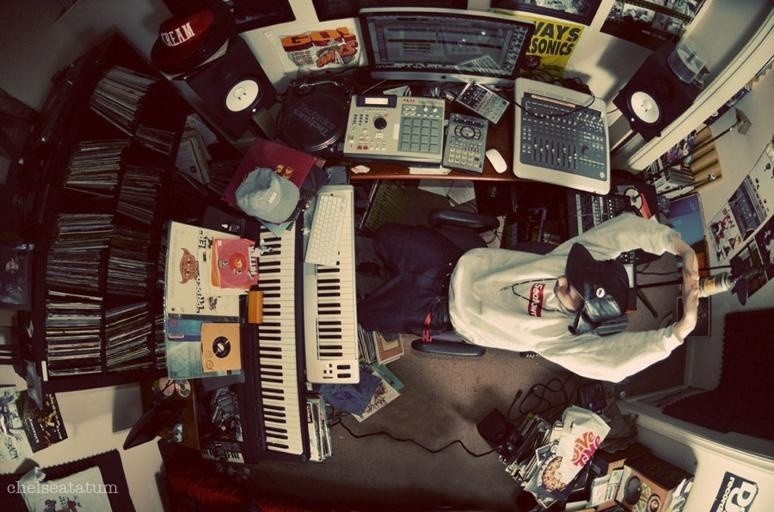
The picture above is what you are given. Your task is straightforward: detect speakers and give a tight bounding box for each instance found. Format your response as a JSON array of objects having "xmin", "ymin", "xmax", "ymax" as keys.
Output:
[
  {"xmin": 172, "ymin": 33, "xmax": 278, "ymax": 145},
  {"xmin": 613, "ymin": 50, "xmax": 704, "ymax": 141},
  {"xmin": 614, "ymin": 451, "xmax": 695, "ymax": 511}
]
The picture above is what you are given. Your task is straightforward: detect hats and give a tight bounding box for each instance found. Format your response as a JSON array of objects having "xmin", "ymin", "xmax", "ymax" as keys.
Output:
[
  {"xmin": 234, "ymin": 166, "xmax": 303, "ymax": 226},
  {"xmin": 147, "ymin": 1, "xmax": 237, "ymax": 76},
  {"xmin": 564, "ymin": 241, "xmax": 633, "ymax": 340}
]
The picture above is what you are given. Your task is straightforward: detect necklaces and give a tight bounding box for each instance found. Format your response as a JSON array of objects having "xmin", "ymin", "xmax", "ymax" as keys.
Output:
[{"xmin": 499, "ymin": 274, "xmax": 572, "ymax": 318}]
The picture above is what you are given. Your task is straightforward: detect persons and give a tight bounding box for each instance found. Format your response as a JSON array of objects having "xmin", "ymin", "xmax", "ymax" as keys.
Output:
[{"xmin": 357, "ymin": 214, "xmax": 700, "ymax": 384}]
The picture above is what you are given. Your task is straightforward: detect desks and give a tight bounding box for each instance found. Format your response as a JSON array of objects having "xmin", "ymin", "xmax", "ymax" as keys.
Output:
[{"xmin": 328, "ymin": 113, "xmax": 527, "ymax": 228}]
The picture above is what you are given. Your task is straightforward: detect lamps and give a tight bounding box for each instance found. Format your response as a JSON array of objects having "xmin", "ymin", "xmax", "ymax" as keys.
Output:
[
  {"xmin": 625, "ymin": 106, "xmax": 752, "ymax": 213},
  {"xmin": 631, "ymin": 92, "xmax": 659, "ymax": 126}
]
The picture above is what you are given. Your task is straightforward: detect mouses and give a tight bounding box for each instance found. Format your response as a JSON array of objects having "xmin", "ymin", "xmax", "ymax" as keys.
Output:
[{"xmin": 486, "ymin": 148, "xmax": 508, "ymax": 174}]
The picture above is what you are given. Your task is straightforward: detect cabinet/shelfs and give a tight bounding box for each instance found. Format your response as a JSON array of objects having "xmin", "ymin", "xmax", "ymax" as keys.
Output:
[{"xmin": 30, "ymin": 25, "xmax": 244, "ymax": 391}]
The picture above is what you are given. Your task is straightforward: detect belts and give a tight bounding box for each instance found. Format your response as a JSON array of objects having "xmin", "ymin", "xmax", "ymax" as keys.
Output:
[{"xmin": 435, "ymin": 245, "xmax": 468, "ymax": 332}]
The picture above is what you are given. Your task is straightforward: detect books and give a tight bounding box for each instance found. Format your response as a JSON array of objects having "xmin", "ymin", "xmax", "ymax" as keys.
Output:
[
  {"xmin": 44, "ymin": 64, "xmax": 259, "ymax": 384},
  {"xmin": 306, "ymin": 327, "xmax": 406, "ymax": 462}
]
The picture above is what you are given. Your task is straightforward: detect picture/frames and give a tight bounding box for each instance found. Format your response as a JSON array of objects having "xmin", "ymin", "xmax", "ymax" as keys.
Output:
[
  {"xmin": 162, "ymin": 0, "xmax": 297, "ymax": 34},
  {"xmin": 490, "ymin": 0, "xmax": 604, "ymax": 26}
]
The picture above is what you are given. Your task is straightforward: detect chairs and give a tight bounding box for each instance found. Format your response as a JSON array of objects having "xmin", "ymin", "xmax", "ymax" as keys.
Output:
[
  {"xmin": 372, "ymin": 209, "xmax": 558, "ymax": 357},
  {"xmin": 656, "ymin": 125, "xmax": 721, "ymax": 203}
]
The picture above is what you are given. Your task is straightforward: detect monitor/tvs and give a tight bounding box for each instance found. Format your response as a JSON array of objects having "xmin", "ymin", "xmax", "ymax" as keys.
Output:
[{"xmin": 357, "ymin": 5, "xmax": 536, "ymax": 100}]
